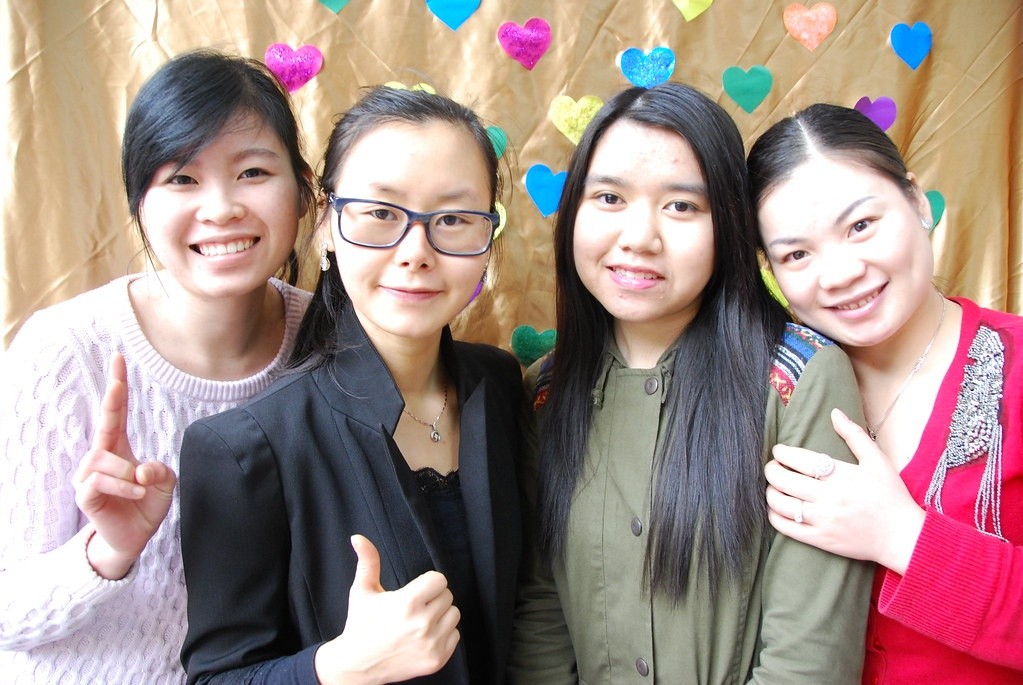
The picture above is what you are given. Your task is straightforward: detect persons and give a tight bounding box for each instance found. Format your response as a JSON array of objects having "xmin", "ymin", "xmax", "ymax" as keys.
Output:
[
  {"xmin": 748, "ymin": 102, "xmax": 1023, "ymax": 685},
  {"xmin": 180, "ymin": 86, "xmax": 536, "ymax": 685},
  {"xmin": 517, "ymin": 82, "xmax": 875, "ymax": 685},
  {"xmin": 0, "ymin": 47, "xmax": 319, "ymax": 685}
]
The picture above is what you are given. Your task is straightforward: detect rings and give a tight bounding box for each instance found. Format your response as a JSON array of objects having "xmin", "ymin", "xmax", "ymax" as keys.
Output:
[
  {"xmin": 815, "ymin": 454, "xmax": 834, "ymax": 478},
  {"xmin": 794, "ymin": 501, "xmax": 803, "ymax": 522}
]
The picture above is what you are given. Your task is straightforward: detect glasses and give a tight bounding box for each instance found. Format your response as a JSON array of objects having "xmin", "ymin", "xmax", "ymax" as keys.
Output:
[{"xmin": 324, "ymin": 187, "xmax": 501, "ymax": 257}]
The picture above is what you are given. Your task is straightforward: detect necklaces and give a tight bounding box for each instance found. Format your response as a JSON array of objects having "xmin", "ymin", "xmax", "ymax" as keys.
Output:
[
  {"xmin": 864, "ymin": 293, "xmax": 945, "ymax": 441},
  {"xmin": 399, "ymin": 383, "xmax": 449, "ymax": 442}
]
